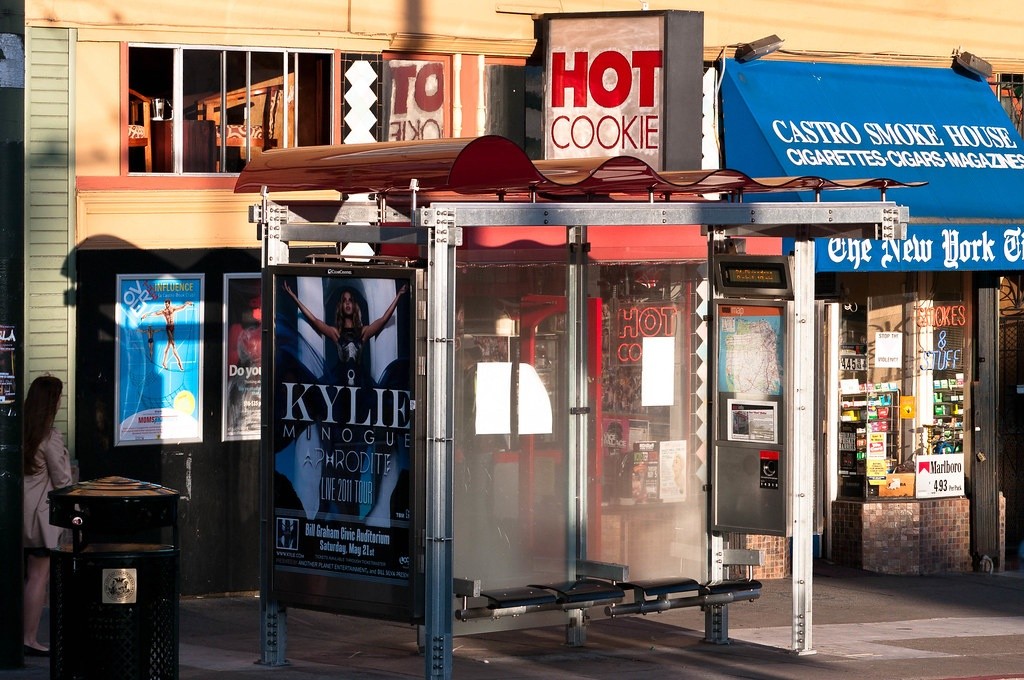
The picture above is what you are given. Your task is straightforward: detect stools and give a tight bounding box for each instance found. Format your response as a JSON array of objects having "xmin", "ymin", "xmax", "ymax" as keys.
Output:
[
  {"xmin": 617, "ymin": 577, "xmax": 701, "ymax": 596},
  {"xmin": 479, "ymin": 586, "xmax": 557, "ymax": 608},
  {"xmin": 700, "ymin": 579, "xmax": 763, "ymax": 595},
  {"xmin": 529, "ymin": 580, "xmax": 627, "ymax": 605}
]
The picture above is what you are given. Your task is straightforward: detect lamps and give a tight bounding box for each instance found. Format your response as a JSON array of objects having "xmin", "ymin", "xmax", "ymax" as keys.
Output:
[
  {"xmin": 956, "ymin": 51, "xmax": 992, "ymax": 78},
  {"xmin": 727, "ymin": 33, "xmax": 786, "ymax": 63},
  {"xmin": 341, "ymin": 242, "xmax": 375, "ymax": 263}
]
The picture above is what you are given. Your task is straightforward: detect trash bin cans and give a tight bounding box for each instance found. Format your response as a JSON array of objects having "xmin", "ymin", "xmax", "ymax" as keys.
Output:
[{"xmin": 46, "ymin": 475, "xmax": 183, "ymax": 680}]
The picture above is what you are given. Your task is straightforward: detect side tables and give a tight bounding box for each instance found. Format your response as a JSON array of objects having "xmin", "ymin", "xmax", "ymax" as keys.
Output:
[{"xmin": 150, "ymin": 119, "xmax": 217, "ymax": 173}]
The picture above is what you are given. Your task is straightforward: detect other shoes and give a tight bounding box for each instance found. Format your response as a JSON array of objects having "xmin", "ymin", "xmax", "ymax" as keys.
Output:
[{"xmin": 24, "ymin": 644, "xmax": 50, "ymax": 657}]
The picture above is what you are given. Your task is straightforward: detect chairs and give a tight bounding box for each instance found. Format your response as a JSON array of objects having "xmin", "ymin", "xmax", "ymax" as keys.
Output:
[
  {"xmin": 128, "ymin": 87, "xmax": 152, "ymax": 173},
  {"xmin": 202, "ymin": 83, "xmax": 284, "ymax": 172}
]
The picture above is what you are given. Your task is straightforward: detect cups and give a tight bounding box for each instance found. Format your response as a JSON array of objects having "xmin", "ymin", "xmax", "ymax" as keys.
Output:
[{"xmin": 153, "ymin": 98, "xmax": 165, "ymax": 121}]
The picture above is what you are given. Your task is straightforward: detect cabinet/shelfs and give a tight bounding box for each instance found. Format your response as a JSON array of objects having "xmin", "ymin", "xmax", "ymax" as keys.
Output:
[
  {"xmin": 838, "ymin": 389, "xmax": 903, "ymax": 478},
  {"xmin": 842, "ymin": 343, "xmax": 869, "ymax": 393},
  {"xmin": 933, "ymin": 367, "xmax": 963, "ymax": 455}
]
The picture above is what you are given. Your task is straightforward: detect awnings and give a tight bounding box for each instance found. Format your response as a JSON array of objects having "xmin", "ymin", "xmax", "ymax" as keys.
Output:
[{"xmin": 720, "ymin": 59, "xmax": 1024, "ymax": 272}]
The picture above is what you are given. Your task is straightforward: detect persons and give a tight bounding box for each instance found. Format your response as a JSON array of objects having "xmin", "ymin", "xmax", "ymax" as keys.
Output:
[
  {"xmin": 142, "ymin": 300, "xmax": 194, "ymax": 345},
  {"xmin": 279, "ymin": 283, "xmax": 407, "ymax": 516},
  {"xmin": 22, "ymin": 377, "xmax": 79, "ymax": 656},
  {"xmin": 136, "ymin": 326, "xmax": 166, "ymax": 360}
]
